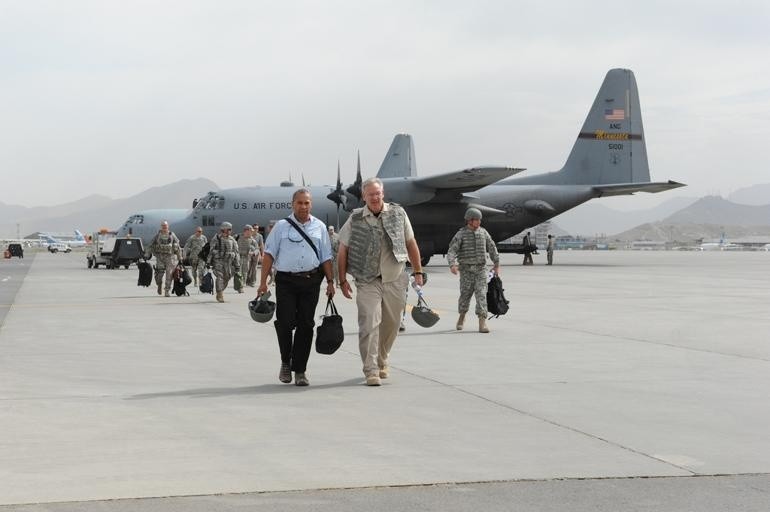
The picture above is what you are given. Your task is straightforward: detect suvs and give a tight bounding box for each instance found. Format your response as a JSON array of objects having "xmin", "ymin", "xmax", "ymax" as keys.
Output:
[{"xmin": 48, "ymin": 242, "xmax": 72, "ymax": 254}]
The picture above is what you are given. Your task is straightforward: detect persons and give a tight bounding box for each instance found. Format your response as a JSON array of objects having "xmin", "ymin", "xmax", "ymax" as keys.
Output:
[
  {"xmin": 246, "ymin": 224, "xmax": 263, "ymax": 287},
  {"xmin": 256, "ymin": 189, "xmax": 334, "ymax": 385},
  {"xmin": 523, "ymin": 231, "xmax": 535, "ymax": 265},
  {"xmin": 446, "ymin": 209, "xmax": 500, "ymax": 332},
  {"xmin": 328, "ymin": 223, "xmax": 339, "ymax": 282},
  {"xmin": 544, "ymin": 234, "xmax": 554, "ymax": 266},
  {"xmin": 182, "ymin": 226, "xmax": 207, "ymax": 287},
  {"xmin": 267, "ymin": 223, "xmax": 275, "ymax": 286},
  {"xmin": 337, "ymin": 177, "xmax": 423, "ymax": 386},
  {"xmin": 234, "ymin": 224, "xmax": 259, "ymax": 293},
  {"xmin": 147, "ymin": 221, "xmax": 182, "ymax": 296},
  {"xmin": 206, "ymin": 221, "xmax": 240, "ymax": 302}
]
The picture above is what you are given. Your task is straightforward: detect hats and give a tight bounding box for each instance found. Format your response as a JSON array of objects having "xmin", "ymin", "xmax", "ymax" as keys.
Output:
[{"xmin": 244, "ymin": 224, "xmax": 253, "ymax": 230}]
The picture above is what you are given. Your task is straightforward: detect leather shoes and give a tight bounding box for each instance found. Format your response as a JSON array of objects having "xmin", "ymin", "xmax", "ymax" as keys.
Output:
[{"xmin": 238, "ymin": 288, "xmax": 245, "ymax": 294}]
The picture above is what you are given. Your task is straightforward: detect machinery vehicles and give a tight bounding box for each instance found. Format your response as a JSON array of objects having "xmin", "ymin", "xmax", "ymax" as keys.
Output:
[{"xmin": 86, "ymin": 233, "xmax": 145, "ymax": 269}]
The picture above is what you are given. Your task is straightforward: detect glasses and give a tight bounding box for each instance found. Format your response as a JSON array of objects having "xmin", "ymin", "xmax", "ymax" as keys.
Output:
[{"xmin": 288, "ymin": 231, "xmax": 305, "ymax": 243}]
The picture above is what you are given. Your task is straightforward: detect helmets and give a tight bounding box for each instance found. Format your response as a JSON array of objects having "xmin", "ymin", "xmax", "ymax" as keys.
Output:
[
  {"xmin": 248, "ymin": 290, "xmax": 276, "ymax": 323},
  {"xmin": 412, "ymin": 307, "xmax": 440, "ymax": 328},
  {"xmin": 464, "ymin": 207, "xmax": 483, "ymax": 222},
  {"xmin": 220, "ymin": 222, "xmax": 233, "ymax": 230}
]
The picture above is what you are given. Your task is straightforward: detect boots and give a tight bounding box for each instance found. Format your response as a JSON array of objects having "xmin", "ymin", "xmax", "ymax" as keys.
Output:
[
  {"xmin": 166, "ymin": 291, "xmax": 171, "ymax": 297},
  {"xmin": 480, "ymin": 317, "xmax": 491, "ymax": 333},
  {"xmin": 456, "ymin": 312, "xmax": 466, "ymax": 331},
  {"xmin": 216, "ymin": 292, "xmax": 225, "ymax": 303},
  {"xmin": 159, "ymin": 286, "xmax": 162, "ymax": 295}
]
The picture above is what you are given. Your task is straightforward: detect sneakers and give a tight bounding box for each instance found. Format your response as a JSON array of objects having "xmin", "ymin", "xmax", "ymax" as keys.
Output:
[
  {"xmin": 380, "ymin": 365, "xmax": 390, "ymax": 378},
  {"xmin": 296, "ymin": 373, "xmax": 310, "ymax": 386},
  {"xmin": 367, "ymin": 375, "xmax": 382, "ymax": 386},
  {"xmin": 280, "ymin": 365, "xmax": 293, "ymax": 384}
]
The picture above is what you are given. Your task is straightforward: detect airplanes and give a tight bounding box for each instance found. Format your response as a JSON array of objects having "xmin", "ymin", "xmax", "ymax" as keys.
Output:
[
  {"xmin": 150, "ymin": 67, "xmax": 688, "ymax": 290},
  {"xmin": 116, "ymin": 134, "xmax": 418, "ymax": 266}
]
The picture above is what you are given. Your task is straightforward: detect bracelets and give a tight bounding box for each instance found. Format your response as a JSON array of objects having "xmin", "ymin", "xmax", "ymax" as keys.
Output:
[
  {"xmin": 338, "ymin": 280, "xmax": 349, "ymax": 286},
  {"xmin": 411, "ymin": 271, "xmax": 424, "ymax": 277},
  {"xmin": 326, "ymin": 280, "xmax": 334, "ymax": 282}
]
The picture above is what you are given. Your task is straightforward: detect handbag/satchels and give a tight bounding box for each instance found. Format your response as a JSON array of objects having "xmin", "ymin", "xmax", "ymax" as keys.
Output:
[
  {"xmin": 314, "ymin": 296, "xmax": 345, "ymax": 355},
  {"xmin": 172, "ymin": 264, "xmax": 192, "ymax": 297}
]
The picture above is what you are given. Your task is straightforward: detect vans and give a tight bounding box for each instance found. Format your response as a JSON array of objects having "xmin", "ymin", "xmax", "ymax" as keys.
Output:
[{"xmin": 6, "ymin": 243, "xmax": 24, "ymax": 258}]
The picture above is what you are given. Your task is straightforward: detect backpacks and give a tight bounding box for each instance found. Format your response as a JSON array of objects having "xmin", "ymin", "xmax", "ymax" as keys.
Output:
[
  {"xmin": 197, "ymin": 235, "xmax": 222, "ymax": 264},
  {"xmin": 199, "ymin": 268, "xmax": 215, "ymax": 296},
  {"xmin": 136, "ymin": 260, "xmax": 153, "ymax": 288},
  {"xmin": 486, "ymin": 273, "xmax": 510, "ymax": 321}
]
